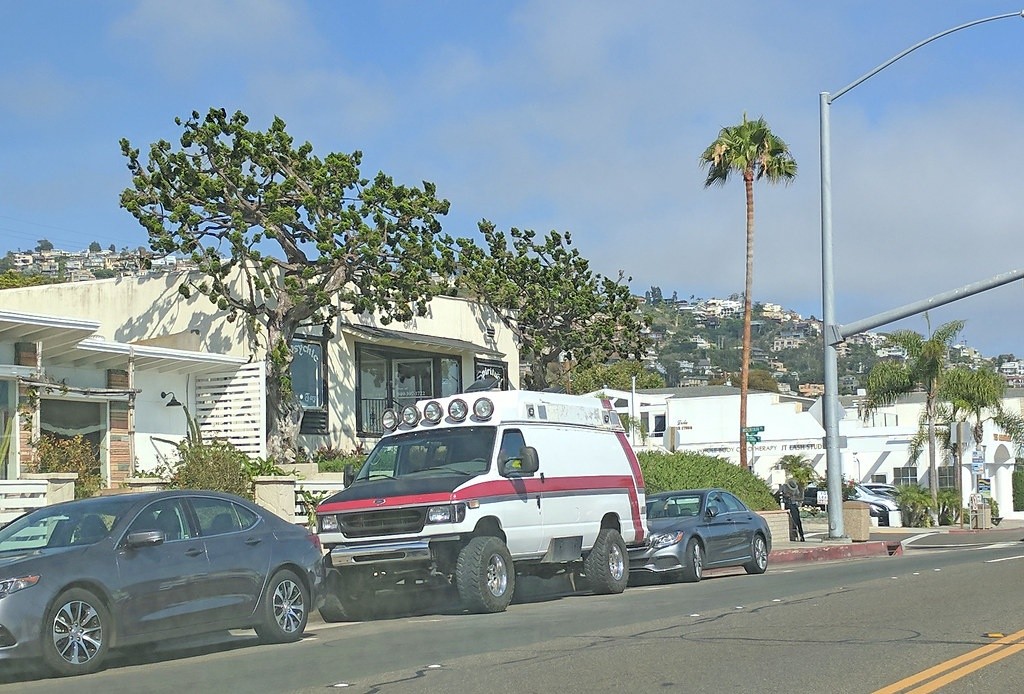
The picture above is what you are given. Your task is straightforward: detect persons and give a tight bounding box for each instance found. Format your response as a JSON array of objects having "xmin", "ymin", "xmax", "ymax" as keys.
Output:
[
  {"xmin": 782, "ymin": 482, "xmax": 805, "ymax": 541},
  {"xmin": 486, "ymin": 434, "xmax": 520, "ymax": 468}
]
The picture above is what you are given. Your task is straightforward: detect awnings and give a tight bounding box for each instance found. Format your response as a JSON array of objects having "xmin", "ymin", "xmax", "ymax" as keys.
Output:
[{"xmin": 347, "ymin": 322, "xmax": 506, "ymax": 359}]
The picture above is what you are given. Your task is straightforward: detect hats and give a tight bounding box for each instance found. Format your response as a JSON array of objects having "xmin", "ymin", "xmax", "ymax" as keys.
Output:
[{"xmin": 786, "ymin": 480, "xmax": 799, "ymax": 490}]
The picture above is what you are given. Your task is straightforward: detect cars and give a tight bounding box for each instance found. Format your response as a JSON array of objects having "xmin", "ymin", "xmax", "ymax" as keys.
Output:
[
  {"xmin": 628, "ymin": 487, "xmax": 773, "ymax": 586},
  {"xmin": 0, "ymin": 491, "xmax": 328, "ymax": 678},
  {"xmin": 804, "ymin": 482, "xmax": 904, "ymax": 525}
]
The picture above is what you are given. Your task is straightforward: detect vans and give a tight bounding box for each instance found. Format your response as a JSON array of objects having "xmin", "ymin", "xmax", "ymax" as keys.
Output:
[{"xmin": 314, "ymin": 391, "xmax": 649, "ymax": 622}]
